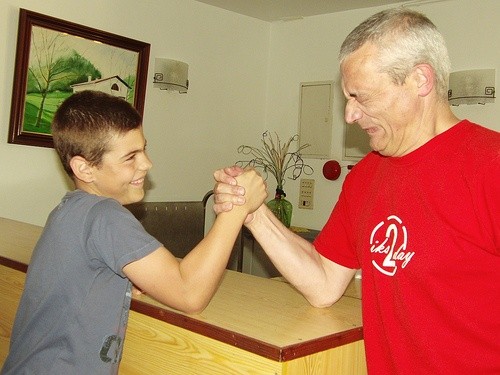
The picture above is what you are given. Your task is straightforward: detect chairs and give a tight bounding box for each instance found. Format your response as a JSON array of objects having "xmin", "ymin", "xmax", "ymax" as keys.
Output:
[{"xmin": 122, "ymin": 190, "xmax": 244, "ymax": 273}]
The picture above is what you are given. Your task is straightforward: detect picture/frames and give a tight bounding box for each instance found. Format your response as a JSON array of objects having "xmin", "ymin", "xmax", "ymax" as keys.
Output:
[{"xmin": 6, "ymin": 8, "xmax": 151, "ymax": 149}]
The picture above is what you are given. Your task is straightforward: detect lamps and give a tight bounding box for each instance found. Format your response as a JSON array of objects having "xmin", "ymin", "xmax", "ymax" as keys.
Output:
[
  {"xmin": 152, "ymin": 57, "xmax": 189, "ymax": 95},
  {"xmin": 448, "ymin": 68, "xmax": 496, "ymax": 107}
]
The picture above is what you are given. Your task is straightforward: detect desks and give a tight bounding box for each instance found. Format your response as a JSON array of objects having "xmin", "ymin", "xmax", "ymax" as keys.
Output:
[{"xmin": 0, "ymin": 215, "xmax": 369, "ymax": 375}]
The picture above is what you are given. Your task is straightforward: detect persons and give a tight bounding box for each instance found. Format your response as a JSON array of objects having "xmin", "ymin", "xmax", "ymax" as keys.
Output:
[
  {"xmin": 0, "ymin": 91, "xmax": 271, "ymax": 375},
  {"xmin": 214, "ymin": 9, "xmax": 500, "ymax": 375}
]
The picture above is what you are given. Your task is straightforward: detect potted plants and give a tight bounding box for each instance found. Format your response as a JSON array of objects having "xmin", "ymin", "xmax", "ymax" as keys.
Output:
[{"xmin": 234, "ymin": 129, "xmax": 315, "ymax": 229}]
